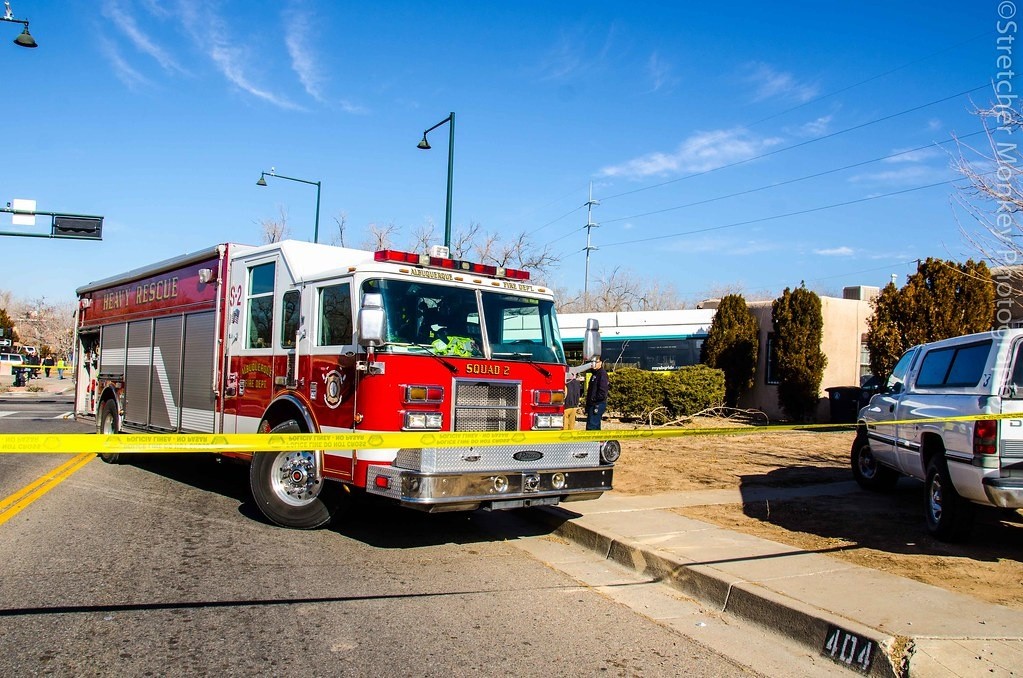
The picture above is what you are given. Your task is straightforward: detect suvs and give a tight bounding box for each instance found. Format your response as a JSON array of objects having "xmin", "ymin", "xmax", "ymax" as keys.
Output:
[{"xmin": 850, "ymin": 328, "xmax": 1023, "ymax": 542}]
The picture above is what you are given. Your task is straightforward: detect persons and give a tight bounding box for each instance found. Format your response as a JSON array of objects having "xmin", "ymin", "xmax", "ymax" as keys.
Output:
[
  {"xmin": 668, "ymin": 356, "xmax": 675, "ymax": 366},
  {"xmin": 418, "ymin": 293, "xmax": 480, "ymax": 342},
  {"xmin": 42, "ymin": 353, "xmax": 54, "ymax": 377},
  {"xmin": 26, "ymin": 352, "xmax": 41, "ymax": 380},
  {"xmin": 56, "ymin": 357, "xmax": 68, "ymax": 380},
  {"xmin": 563, "ymin": 372, "xmax": 580, "ymax": 430},
  {"xmin": 584, "ymin": 355, "xmax": 609, "ymax": 430}
]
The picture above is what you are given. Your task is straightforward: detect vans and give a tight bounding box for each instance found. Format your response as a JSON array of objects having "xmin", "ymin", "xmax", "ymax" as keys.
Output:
[{"xmin": 0, "ymin": 346, "xmax": 38, "ymax": 368}]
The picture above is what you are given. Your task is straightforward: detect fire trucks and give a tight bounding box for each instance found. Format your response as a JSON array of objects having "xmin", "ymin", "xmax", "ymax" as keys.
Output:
[{"xmin": 72, "ymin": 238, "xmax": 622, "ymax": 529}]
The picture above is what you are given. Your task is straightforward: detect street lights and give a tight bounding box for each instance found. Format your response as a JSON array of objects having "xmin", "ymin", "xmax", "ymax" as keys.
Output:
[
  {"xmin": 256, "ymin": 171, "xmax": 322, "ymax": 244},
  {"xmin": 416, "ymin": 112, "xmax": 456, "ymax": 259}
]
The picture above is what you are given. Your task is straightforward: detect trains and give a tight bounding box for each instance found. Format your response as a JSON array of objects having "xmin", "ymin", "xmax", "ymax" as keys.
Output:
[{"xmin": 467, "ymin": 309, "xmax": 719, "ymax": 371}]
